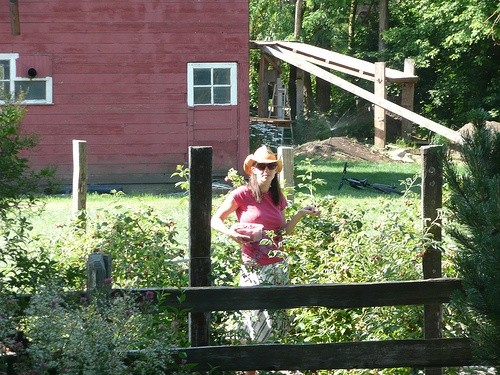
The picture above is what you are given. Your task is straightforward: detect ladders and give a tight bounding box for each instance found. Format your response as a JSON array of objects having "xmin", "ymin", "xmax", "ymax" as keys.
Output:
[{"xmin": 260, "ymin": 83, "xmax": 295, "ymax": 157}]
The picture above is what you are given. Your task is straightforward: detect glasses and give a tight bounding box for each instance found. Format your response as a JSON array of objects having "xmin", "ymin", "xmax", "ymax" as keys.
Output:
[{"xmin": 254, "ymin": 163, "xmax": 279, "ymax": 170}]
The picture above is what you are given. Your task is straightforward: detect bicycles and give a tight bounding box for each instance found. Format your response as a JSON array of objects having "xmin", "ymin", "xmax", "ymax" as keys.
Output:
[{"xmin": 338, "ymin": 163, "xmax": 404, "ymax": 195}]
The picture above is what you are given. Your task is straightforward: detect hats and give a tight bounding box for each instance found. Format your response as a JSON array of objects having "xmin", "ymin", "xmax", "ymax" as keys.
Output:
[{"xmin": 243, "ymin": 146, "xmax": 283, "ymax": 174}]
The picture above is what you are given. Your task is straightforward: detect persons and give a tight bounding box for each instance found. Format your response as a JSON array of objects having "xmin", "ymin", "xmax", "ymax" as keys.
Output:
[{"xmin": 211, "ymin": 149, "xmax": 322, "ymax": 375}]
